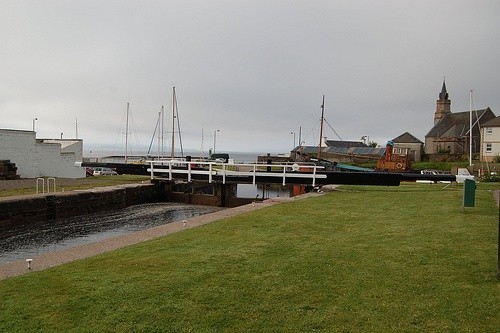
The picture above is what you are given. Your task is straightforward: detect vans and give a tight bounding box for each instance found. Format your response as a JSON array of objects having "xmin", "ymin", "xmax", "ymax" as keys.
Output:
[
  {"xmin": 86, "ymin": 167, "xmax": 94, "ymax": 177},
  {"xmin": 93, "ymin": 166, "xmax": 118, "ymax": 176}
]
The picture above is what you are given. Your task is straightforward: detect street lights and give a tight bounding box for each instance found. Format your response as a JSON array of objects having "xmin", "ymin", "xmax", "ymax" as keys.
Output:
[
  {"xmin": 32, "ymin": 117, "xmax": 38, "ymax": 130},
  {"xmin": 213, "ymin": 128, "xmax": 220, "ymax": 153},
  {"xmin": 290, "ymin": 132, "xmax": 296, "ymax": 149}
]
